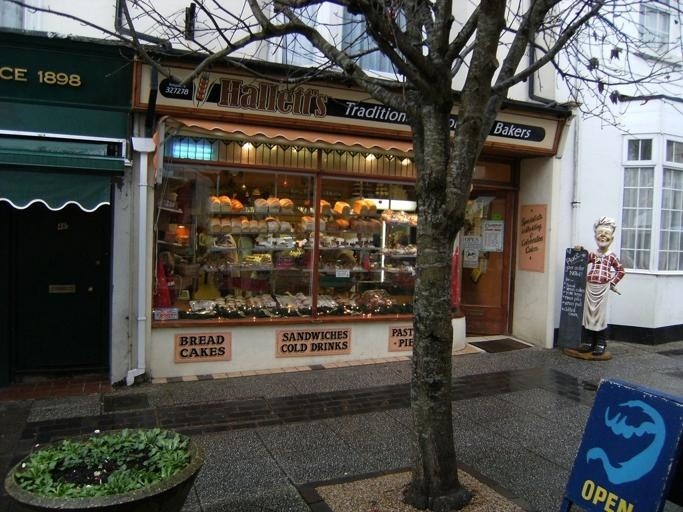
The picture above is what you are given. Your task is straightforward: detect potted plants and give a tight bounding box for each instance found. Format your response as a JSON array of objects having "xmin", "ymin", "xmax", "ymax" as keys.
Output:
[{"xmin": 2, "ymin": 419, "xmax": 205, "ymax": 512}]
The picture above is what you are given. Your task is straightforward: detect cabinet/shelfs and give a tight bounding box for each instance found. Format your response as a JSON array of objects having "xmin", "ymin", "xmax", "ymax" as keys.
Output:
[
  {"xmin": 153, "ymin": 170, "xmax": 196, "ymax": 294},
  {"xmin": 379, "ymin": 214, "xmax": 419, "ymax": 278},
  {"xmin": 197, "ymin": 208, "xmax": 317, "ymax": 274},
  {"xmin": 315, "ymin": 211, "xmax": 382, "ymax": 276}
]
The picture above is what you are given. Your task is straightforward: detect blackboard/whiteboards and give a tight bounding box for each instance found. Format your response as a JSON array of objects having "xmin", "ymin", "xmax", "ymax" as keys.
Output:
[{"xmin": 557, "ymin": 247, "xmax": 589, "ymax": 350}]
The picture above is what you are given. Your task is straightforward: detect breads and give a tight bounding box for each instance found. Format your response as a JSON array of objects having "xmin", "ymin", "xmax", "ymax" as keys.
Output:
[{"xmin": 164, "ymin": 191, "xmax": 417, "ymax": 318}]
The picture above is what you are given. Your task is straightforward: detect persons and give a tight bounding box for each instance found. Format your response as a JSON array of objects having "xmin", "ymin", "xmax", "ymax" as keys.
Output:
[{"xmin": 574, "ymin": 216, "xmax": 625, "ymax": 355}]
